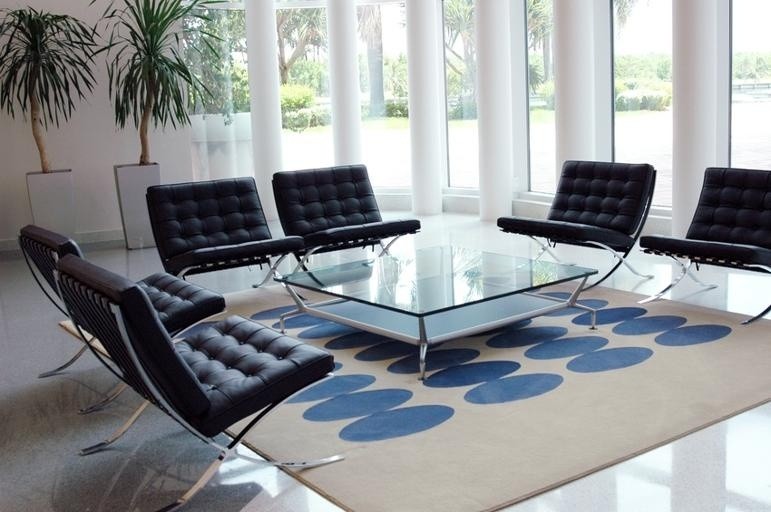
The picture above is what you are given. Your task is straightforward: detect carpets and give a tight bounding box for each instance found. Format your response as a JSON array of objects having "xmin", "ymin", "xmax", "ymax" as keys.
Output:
[{"xmin": 55, "ymin": 280, "xmax": 770, "ymax": 512}]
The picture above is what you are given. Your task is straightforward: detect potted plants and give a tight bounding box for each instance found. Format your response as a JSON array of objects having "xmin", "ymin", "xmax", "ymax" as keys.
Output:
[
  {"xmin": 82, "ymin": 1, "xmax": 226, "ymax": 250},
  {"xmin": 1, "ymin": 5, "xmax": 109, "ymax": 246}
]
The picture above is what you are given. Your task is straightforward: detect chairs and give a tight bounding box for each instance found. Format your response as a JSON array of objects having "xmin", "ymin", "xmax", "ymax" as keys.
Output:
[
  {"xmin": 639, "ymin": 166, "xmax": 771, "ymax": 327},
  {"xmin": 51, "ymin": 256, "xmax": 346, "ymax": 512},
  {"xmin": 137, "ymin": 161, "xmax": 426, "ymax": 298},
  {"xmin": 496, "ymin": 160, "xmax": 659, "ymax": 295},
  {"xmin": 19, "ymin": 222, "xmax": 228, "ymax": 414}
]
